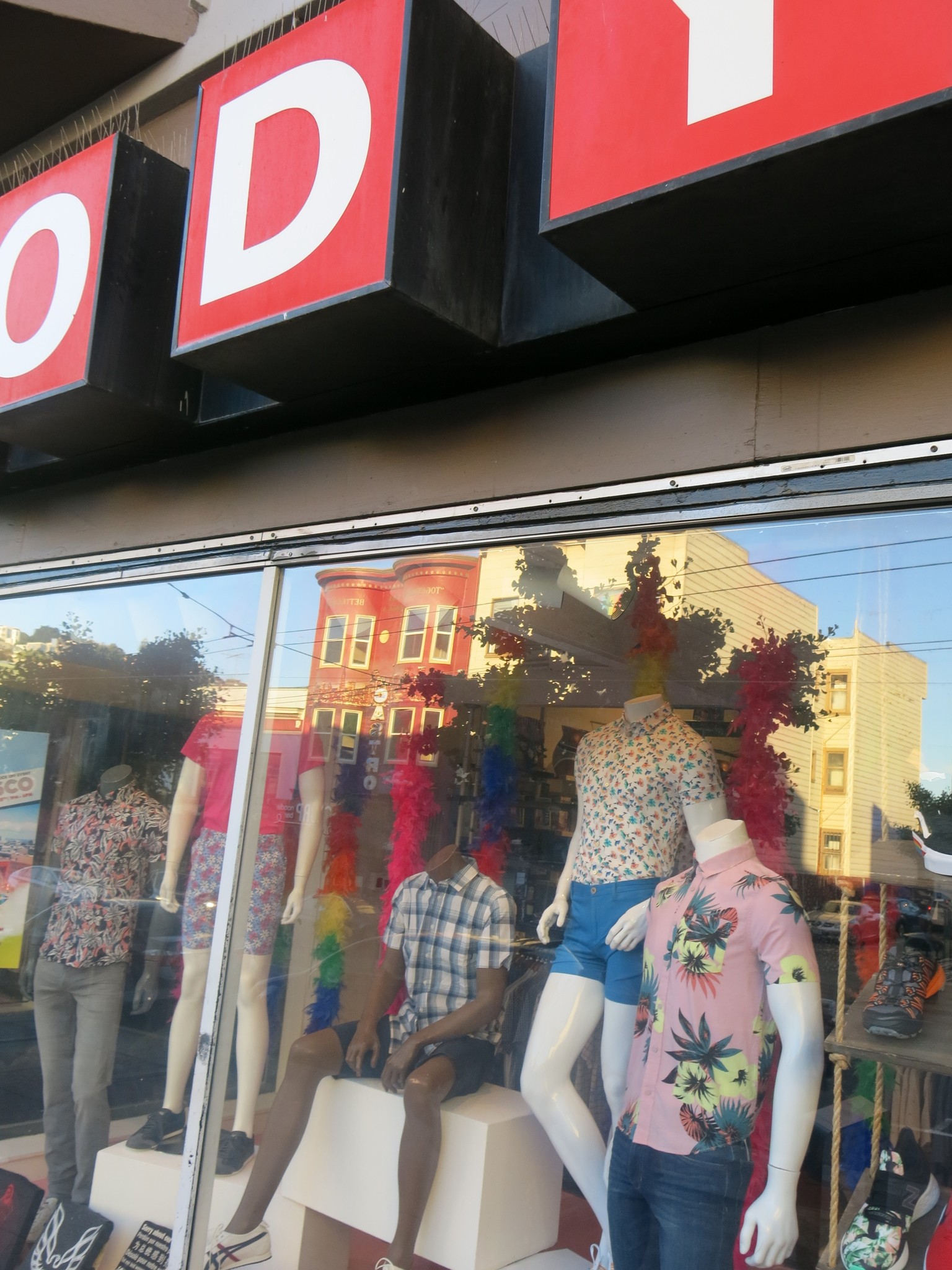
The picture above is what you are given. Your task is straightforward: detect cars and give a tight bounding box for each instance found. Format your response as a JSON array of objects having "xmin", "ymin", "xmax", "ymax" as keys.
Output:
[
  {"xmin": 861, "ymin": 895, "xmax": 933, "ymax": 936},
  {"xmin": 7, "ymin": 864, "xmax": 181, "ymax": 1020},
  {"xmin": 810, "ymin": 900, "xmax": 882, "ymax": 946},
  {"xmin": 928, "ymin": 899, "xmax": 946, "ymax": 926}
]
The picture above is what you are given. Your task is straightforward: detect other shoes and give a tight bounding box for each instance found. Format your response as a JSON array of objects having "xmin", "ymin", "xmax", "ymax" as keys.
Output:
[
  {"xmin": 25, "ymin": 1197, "xmax": 61, "ymax": 1245},
  {"xmin": 923, "ymin": 1195, "xmax": 952, "ymax": 1270},
  {"xmin": 911, "ymin": 771, "xmax": 952, "ymax": 876}
]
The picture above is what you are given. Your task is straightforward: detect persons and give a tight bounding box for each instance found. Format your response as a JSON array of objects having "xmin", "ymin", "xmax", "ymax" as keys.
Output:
[
  {"xmin": 18, "ymin": 765, "xmax": 176, "ymax": 1243},
  {"xmin": 520, "ymin": 693, "xmax": 730, "ymax": 1270},
  {"xmin": 126, "ymin": 708, "xmax": 331, "ymax": 1174},
  {"xmin": 201, "ymin": 843, "xmax": 518, "ymax": 1270},
  {"xmin": 606, "ymin": 818, "xmax": 824, "ymax": 1270}
]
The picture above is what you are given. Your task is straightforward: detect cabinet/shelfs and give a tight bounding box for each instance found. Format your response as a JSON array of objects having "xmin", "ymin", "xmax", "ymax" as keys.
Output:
[{"xmin": 816, "ymin": 548, "xmax": 951, "ymax": 1270}]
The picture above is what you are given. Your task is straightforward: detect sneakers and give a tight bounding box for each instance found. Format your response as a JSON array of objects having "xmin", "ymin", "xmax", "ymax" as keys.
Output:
[
  {"xmin": 861, "ymin": 939, "xmax": 946, "ymax": 1039},
  {"xmin": 842, "ymin": 1124, "xmax": 942, "ymax": 1270},
  {"xmin": 216, "ymin": 1131, "xmax": 256, "ymax": 1176},
  {"xmin": 586, "ymin": 1243, "xmax": 615, "ymax": 1270},
  {"xmin": 203, "ymin": 1220, "xmax": 272, "ymax": 1270},
  {"xmin": 375, "ymin": 1257, "xmax": 406, "ymax": 1270},
  {"xmin": 124, "ymin": 1107, "xmax": 186, "ymax": 1152}
]
